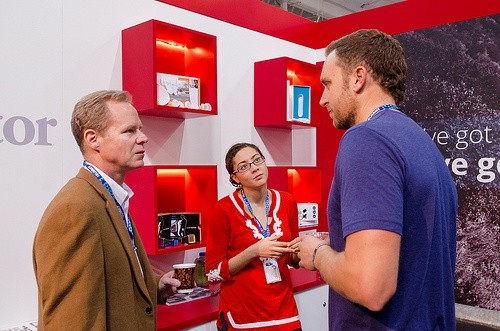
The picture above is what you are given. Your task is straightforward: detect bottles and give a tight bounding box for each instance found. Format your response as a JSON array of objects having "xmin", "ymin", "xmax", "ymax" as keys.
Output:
[{"xmin": 194, "ymin": 252, "xmax": 210, "ymax": 287}]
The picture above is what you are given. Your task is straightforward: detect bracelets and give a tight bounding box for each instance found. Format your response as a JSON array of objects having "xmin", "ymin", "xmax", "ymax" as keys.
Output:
[{"xmin": 312, "ymin": 243, "xmax": 329, "ymax": 267}]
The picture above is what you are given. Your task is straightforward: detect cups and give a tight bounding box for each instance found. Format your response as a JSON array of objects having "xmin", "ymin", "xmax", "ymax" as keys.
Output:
[
  {"xmin": 311, "ymin": 232, "xmax": 329, "ymax": 242},
  {"xmin": 173, "ymin": 263, "xmax": 196, "ymax": 293}
]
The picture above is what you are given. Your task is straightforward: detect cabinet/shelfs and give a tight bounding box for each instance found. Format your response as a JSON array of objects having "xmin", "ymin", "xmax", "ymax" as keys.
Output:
[
  {"xmin": 267, "ymin": 164, "xmax": 323, "ymax": 230},
  {"xmin": 121, "ymin": 19, "xmax": 217, "ymax": 119},
  {"xmin": 123, "ymin": 164, "xmax": 218, "ymax": 255},
  {"xmin": 154, "ymin": 262, "xmax": 332, "ymax": 331},
  {"xmin": 253, "ymin": 57, "xmax": 325, "ymax": 127}
]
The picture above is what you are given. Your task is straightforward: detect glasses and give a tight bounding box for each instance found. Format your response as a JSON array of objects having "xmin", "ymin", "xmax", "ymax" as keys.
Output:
[{"xmin": 233, "ymin": 155, "xmax": 265, "ymax": 173}]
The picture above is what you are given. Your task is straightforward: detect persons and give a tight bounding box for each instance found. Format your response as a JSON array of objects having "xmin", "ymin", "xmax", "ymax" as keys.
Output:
[
  {"xmin": 204, "ymin": 143, "xmax": 302, "ymax": 331},
  {"xmin": 298, "ymin": 30, "xmax": 457, "ymax": 331},
  {"xmin": 33, "ymin": 91, "xmax": 197, "ymax": 331}
]
